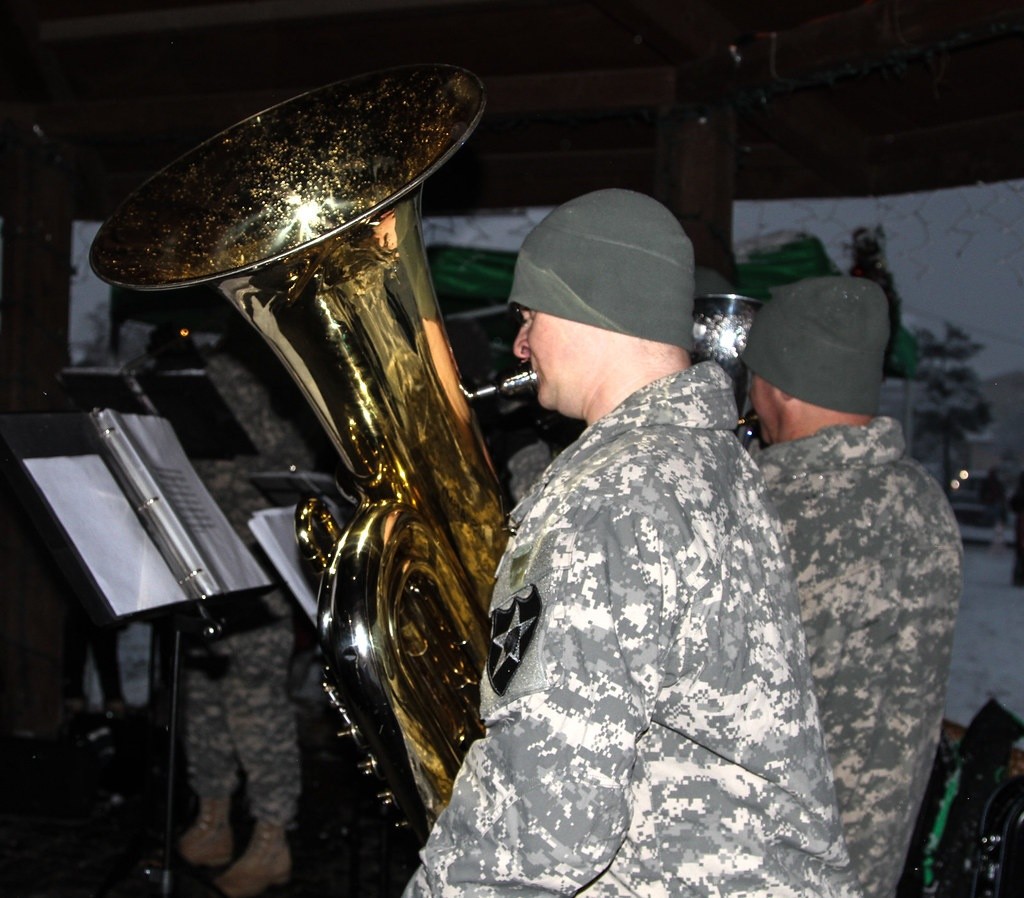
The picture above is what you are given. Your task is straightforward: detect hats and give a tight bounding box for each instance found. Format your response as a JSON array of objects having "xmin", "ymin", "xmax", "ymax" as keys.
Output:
[
  {"xmin": 694, "ymin": 264, "xmax": 735, "ymax": 299},
  {"xmin": 744, "ymin": 276, "xmax": 891, "ymax": 414},
  {"xmin": 509, "ymin": 188, "xmax": 695, "ymax": 351}
]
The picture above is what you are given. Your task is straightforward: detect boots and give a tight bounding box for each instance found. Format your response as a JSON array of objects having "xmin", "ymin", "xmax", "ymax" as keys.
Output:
[
  {"xmin": 213, "ymin": 821, "xmax": 293, "ymax": 897},
  {"xmin": 175, "ymin": 797, "xmax": 232, "ymax": 864}
]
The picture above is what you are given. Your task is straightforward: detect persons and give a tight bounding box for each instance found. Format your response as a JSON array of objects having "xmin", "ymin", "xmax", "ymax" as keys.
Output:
[
  {"xmin": 402, "ymin": 190, "xmax": 859, "ymax": 897},
  {"xmin": 181, "ymin": 334, "xmax": 302, "ymax": 898},
  {"xmin": 739, "ymin": 277, "xmax": 964, "ymax": 896}
]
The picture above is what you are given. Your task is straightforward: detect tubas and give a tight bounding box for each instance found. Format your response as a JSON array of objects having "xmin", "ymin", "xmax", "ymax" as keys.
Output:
[{"xmin": 86, "ymin": 61, "xmax": 540, "ymax": 846}]
